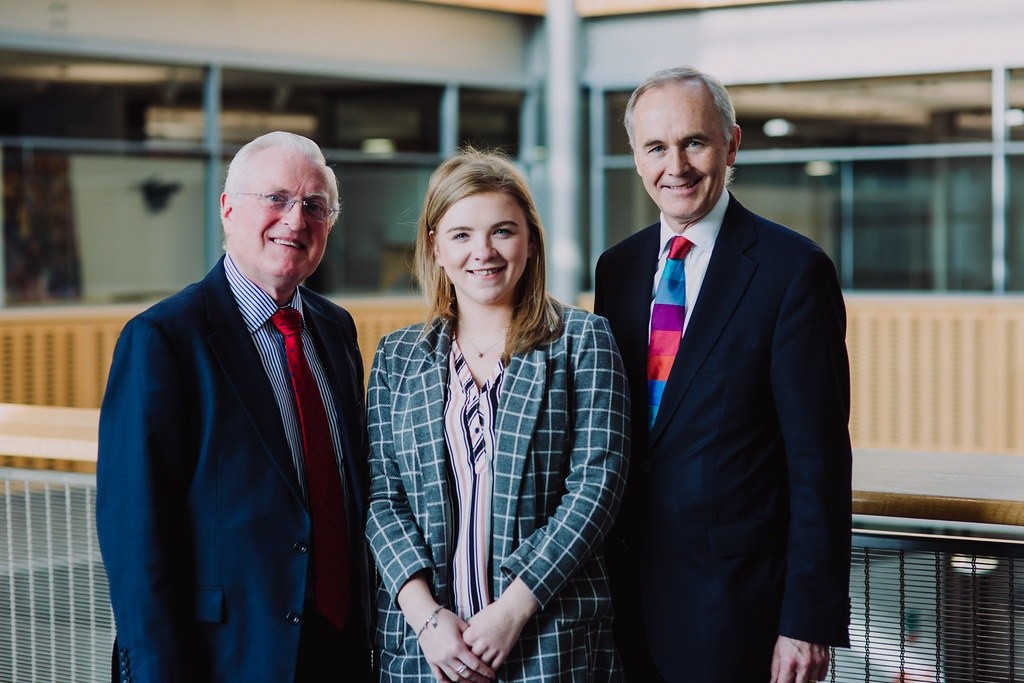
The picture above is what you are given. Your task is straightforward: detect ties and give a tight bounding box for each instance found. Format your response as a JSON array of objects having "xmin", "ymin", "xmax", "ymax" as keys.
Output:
[
  {"xmin": 271, "ymin": 306, "xmax": 351, "ymax": 630},
  {"xmin": 647, "ymin": 236, "xmax": 695, "ymax": 435}
]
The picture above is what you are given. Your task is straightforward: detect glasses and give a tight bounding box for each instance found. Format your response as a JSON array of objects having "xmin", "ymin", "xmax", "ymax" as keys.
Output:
[{"xmin": 228, "ymin": 192, "xmax": 338, "ymax": 220}]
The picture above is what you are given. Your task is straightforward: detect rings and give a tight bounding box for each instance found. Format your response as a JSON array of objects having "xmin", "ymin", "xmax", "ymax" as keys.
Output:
[
  {"xmin": 456, "ymin": 664, "xmax": 467, "ymax": 675},
  {"xmin": 809, "ymin": 678, "xmax": 820, "ymax": 683}
]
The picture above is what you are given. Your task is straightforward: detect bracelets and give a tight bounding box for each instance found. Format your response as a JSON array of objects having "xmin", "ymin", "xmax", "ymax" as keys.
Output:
[{"xmin": 416, "ymin": 605, "xmax": 444, "ymax": 641}]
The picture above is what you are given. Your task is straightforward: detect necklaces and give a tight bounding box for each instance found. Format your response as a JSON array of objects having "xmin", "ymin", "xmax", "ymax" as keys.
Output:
[{"xmin": 456, "ymin": 318, "xmax": 505, "ymax": 356}]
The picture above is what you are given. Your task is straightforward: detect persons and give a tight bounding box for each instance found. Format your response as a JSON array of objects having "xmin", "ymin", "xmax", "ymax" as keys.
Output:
[
  {"xmin": 365, "ymin": 148, "xmax": 632, "ymax": 683},
  {"xmin": 96, "ymin": 131, "xmax": 368, "ymax": 683},
  {"xmin": 593, "ymin": 65, "xmax": 852, "ymax": 683}
]
